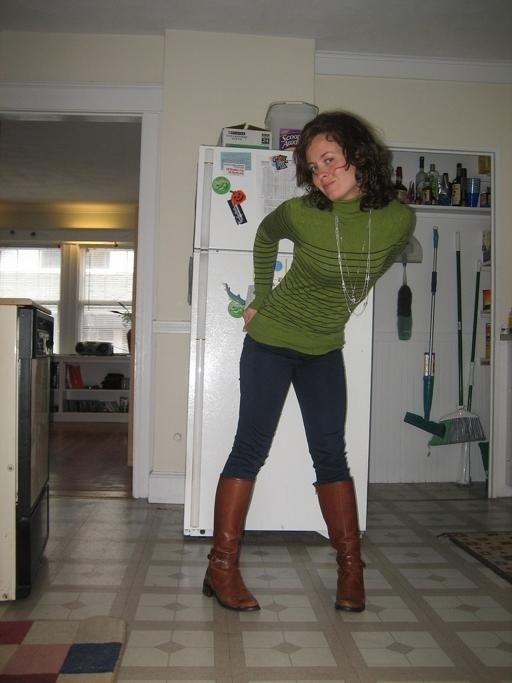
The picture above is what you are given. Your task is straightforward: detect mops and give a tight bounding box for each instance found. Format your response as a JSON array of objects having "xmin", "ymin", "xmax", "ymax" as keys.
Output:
[{"xmin": 402, "ymin": 225, "xmax": 447, "ymax": 439}]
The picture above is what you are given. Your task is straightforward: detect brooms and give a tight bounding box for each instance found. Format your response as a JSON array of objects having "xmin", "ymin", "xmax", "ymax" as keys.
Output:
[{"xmin": 427, "ymin": 230, "xmax": 486, "ymax": 448}]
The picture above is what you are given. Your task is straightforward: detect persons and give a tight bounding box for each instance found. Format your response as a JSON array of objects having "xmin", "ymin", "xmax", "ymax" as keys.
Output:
[{"xmin": 202, "ymin": 112, "xmax": 416, "ymax": 611}]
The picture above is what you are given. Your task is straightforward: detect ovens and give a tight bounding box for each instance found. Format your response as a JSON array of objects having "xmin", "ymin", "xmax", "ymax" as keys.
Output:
[{"xmin": 17, "ymin": 309, "xmax": 56, "ymax": 597}]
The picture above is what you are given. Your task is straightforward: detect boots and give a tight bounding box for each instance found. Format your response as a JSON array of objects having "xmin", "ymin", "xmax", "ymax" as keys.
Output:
[
  {"xmin": 200, "ymin": 470, "xmax": 264, "ymax": 618},
  {"xmin": 309, "ymin": 473, "xmax": 368, "ymax": 616}
]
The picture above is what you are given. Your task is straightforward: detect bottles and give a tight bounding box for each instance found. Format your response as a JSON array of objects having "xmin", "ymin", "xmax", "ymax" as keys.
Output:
[{"xmin": 393, "ymin": 155, "xmax": 467, "ymax": 207}]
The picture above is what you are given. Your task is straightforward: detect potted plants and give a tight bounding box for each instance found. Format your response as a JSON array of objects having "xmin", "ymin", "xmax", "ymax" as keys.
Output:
[{"xmin": 111, "ymin": 301, "xmax": 133, "ymax": 353}]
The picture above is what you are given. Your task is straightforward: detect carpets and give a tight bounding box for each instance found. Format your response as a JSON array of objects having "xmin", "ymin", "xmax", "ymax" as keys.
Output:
[
  {"xmin": 438, "ymin": 529, "xmax": 511, "ymax": 584},
  {"xmin": 1, "ymin": 618, "xmax": 129, "ymax": 683}
]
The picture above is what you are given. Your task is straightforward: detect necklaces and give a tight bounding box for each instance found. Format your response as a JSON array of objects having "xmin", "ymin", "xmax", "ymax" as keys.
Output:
[{"xmin": 335, "ymin": 207, "xmax": 372, "ymax": 316}]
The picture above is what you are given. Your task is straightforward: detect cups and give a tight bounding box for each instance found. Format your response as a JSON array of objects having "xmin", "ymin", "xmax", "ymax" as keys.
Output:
[
  {"xmin": 120, "ymin": 397, "xmax": 129, "ymax": 413},
  {"xmin": 120, "ymin": 378, "xmax": 130, "ymax": 390},
  {"xmin": 465, "ymin": 177, "xmax": 481, "ymax": 208}
]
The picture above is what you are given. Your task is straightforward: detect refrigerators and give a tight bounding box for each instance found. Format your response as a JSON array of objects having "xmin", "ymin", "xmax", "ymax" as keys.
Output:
[{"xmin": 181, "ymin": 144, "xmax": 378, "ymax": 542}]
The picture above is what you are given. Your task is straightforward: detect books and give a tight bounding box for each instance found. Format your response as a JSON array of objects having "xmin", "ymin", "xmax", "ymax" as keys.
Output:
[
  {"xmin": 62, "ymin": 398, "xmax": 129, "ymax": 413},
  {"xmin": 66, "ymin": 365, "xmax": 84, "ymax": 389}
]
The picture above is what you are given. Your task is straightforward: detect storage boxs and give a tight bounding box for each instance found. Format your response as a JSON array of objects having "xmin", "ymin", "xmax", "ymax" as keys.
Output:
[
  {"xmin": 264, "ymin": 100, "xmax": 318, "ymax": 150},
  {"xmin": 217, "ymin": 122, "xmax": 272, "ymax": 149}
]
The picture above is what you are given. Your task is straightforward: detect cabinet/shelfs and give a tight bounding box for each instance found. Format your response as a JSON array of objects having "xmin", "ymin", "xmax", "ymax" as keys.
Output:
[{"xmin": 51, "ymin": 353, "xmax": 133, "ymax": 424}]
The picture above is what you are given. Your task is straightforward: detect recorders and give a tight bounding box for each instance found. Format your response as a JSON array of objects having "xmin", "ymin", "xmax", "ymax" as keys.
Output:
[{"xmin": 74, "ymin": 341, "xmax": 113, "ymax": 355}]
